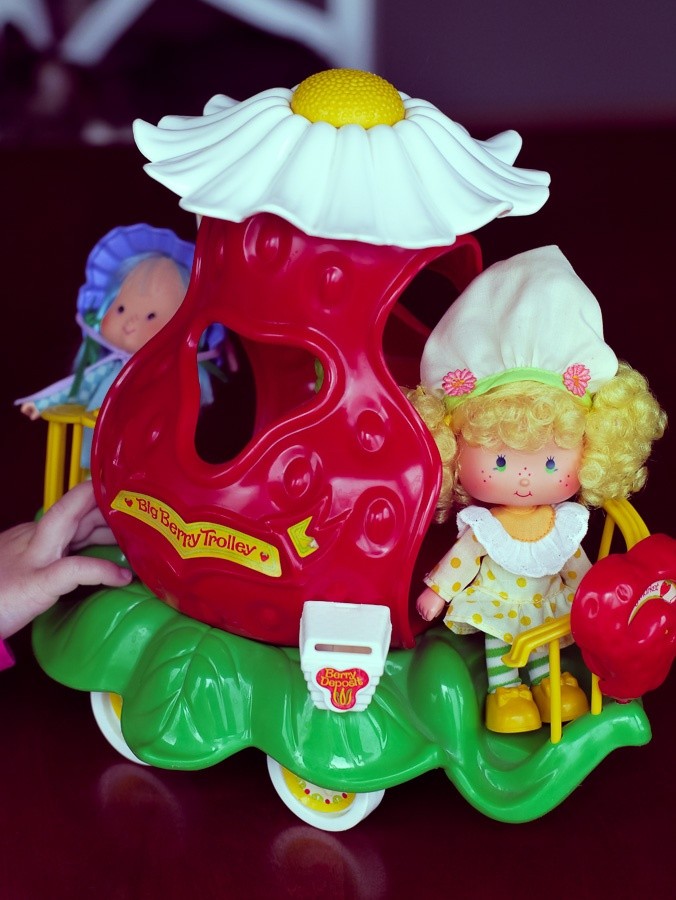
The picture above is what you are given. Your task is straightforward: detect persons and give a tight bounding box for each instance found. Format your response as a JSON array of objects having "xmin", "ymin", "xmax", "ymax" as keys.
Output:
[{"xmin": 0, "ymin": 476, "xmax": 134, "ymax": 672}]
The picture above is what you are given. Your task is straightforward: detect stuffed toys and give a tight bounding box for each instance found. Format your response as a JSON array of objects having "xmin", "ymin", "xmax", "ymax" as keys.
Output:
[
  {"xmin": 378, "ymin": 243, "xmax": 669, "ymax": 736},
  {"xmin": 16, "ymin": 222, "xmax": 225, "ymax": 483}
]
